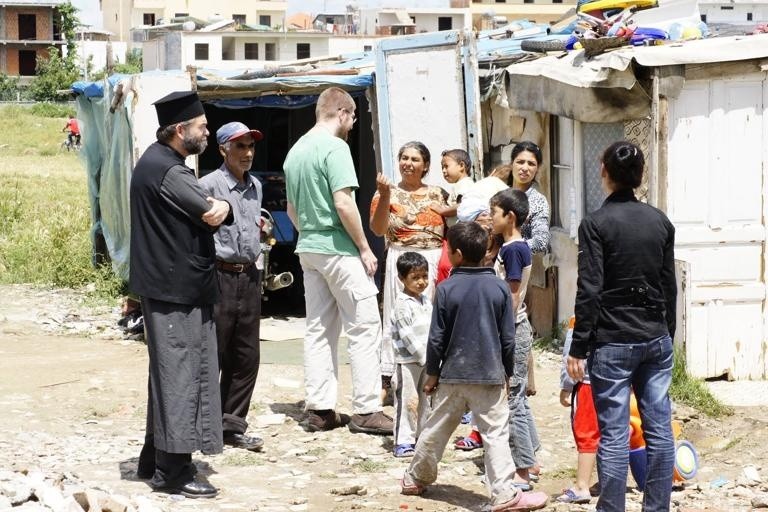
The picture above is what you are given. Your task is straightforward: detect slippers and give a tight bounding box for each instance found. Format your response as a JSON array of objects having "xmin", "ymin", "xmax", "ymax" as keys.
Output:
[
  {"xmin": 391, "ymin": 444, "xmax": 416, "ymax": 457},
  {"xmin": 398, "ymin": 475, "xmax": 424, "ymax": 495},
  {"xmin": 488, "ymin": 490, "xmax": 549, "ymax": 512},
  {"xmin": 482, "ymin": 472, "xmax": 539, "ymax": 489},
  {"xmin": 455, "ymin": 437, "xmax": 484, "ymax": 450},
  {"xmin": 556, "ymin": 488, "xmax": 593, "ymax": 504}
]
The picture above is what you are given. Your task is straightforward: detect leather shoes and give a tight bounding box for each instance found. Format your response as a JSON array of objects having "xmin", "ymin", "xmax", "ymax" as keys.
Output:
[
  {"xmin": 174, "ymin": 476, "xmax": 217, "ymax": 500},
  {"xmin": 211, "ymin": 260, "xmax": 256, "ymax": 275}
]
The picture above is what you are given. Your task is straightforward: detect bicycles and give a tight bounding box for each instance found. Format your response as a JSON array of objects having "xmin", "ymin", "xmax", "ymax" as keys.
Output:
[
  {"xmin": 58, "ymin": 129, "xmax": 82, "ymax": 153},
  {"xmin": 514, "ymin": 3, "xmax": 641, "ymax": 55}
]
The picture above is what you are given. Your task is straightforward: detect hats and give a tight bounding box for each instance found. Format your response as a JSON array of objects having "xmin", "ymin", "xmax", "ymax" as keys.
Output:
[
  {"xmin": 150, "ymin": 89, "xmax": 206, "ymax": 127},
  {"xmin": 216, "ymin": 121, "xmax": 264, "ymax": 143}
]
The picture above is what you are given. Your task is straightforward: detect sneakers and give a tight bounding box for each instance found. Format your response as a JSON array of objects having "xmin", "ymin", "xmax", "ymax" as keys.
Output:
[
  {"xmin": 349, "ymin": 410, "xmax": 396, "ymax": 433},
  {"xmin": 225, "ymin": 430, "xmax": 266, "ymax": 450},
  {"xmin": 304, "ymin": 409, "xmax": 351, "ymax": 431}
]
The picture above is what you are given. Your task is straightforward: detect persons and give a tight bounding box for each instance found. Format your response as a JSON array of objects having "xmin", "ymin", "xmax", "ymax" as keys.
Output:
[
  {"xmin": 194, "ymin": 119, "xmax": 265, "ymax": 450},
  {"xmin": 457, "ymin": 166, "xmax": 513, "ymax": 225},
  {"xmin": 61, "ymin": 114, "xmax": 81, "ymax": 148},
  {"xmin": 401, "ymin": 222, "xmax": 549, "ymax": 510},
  {"xmin": 489, "ymin": 188, "xmax": 539, "ymax": 491},
  {"xmin": 455, "ymin": 142, "xmax": 550, "ymax": 451},
  {"xmin": 429, "ymin": 149, "xmax": 474, "ymax": 226},
  {"xmin": 566, "ymin": 142, "xmax": 677, "ymax": 510},
  {"xmin": 387, "ymin": 252, "xmax": 434, "ymax": 457},
  {"xmin": 555, "ymin": 311, "xmax": 646, "ymax": 502},
  {"xmin": 370, "ymin": 142, "xmax": 449, "ymax": 404},
  {"xmin": 127, "ymin": 89, "xmax": 239, "ymax": 497},
  {"xmin": 282, "ymin": 87, "xmax": 396, "ymax": 436}
]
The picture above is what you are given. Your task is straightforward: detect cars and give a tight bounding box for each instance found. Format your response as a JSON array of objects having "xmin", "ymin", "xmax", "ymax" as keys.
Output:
[{"xmin": 248, "ymin": 168, "xmax": 297, "ymax": 252}]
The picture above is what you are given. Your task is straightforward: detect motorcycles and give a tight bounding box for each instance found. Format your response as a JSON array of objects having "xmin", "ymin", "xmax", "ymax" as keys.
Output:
[{"xmin": 258, "ymin": 206, "xmax": 296, "ymax": 306}]
